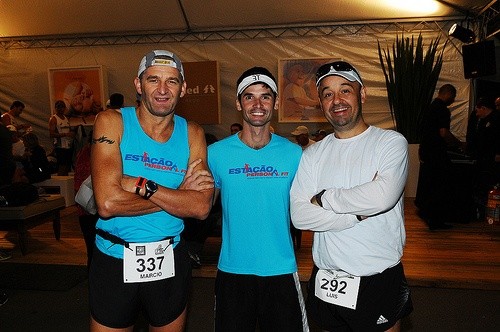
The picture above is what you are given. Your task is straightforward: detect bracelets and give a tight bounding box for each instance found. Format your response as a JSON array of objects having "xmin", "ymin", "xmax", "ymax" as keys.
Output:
[
  {"xmin": 63, "ymin": 133, "xmax": 65, "ymax": 137},
  {"xmin": 136, "ymin": 177, "xmax": 146, "ymax": 195},
  {"xmin": 356, "ymin": 215, "xmax": 362, "ymax": 221}
]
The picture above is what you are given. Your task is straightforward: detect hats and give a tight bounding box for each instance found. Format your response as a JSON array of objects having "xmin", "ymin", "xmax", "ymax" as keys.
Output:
[
  {"xmin": 237, "ymin": 66, "xmax": 279, "ymax": 100},
  {"xmin": 316, "ymin": 61, "xmax": 365, "ymax": 89},
  {"xmin": 138, "ymin": 49, "xmax": 184, "ymax": 83}
]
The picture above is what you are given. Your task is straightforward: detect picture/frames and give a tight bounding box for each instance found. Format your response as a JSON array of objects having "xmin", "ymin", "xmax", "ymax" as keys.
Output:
[
  {"xmin": 47, "ymin": 65, "xmax": 106, "ymax": 127},
  {"xmin": 277, "ymin": 56, "xmax": 343, "ymax": 124}
]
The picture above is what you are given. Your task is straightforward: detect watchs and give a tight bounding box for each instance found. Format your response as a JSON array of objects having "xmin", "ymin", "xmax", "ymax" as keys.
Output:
[
  {"xmin": 142, "ymin": 180, "xmax": 158, "ymax": 200},
  {"xmin": 316, "ymin": 190, "xmax": 326, "ymax": 206}
]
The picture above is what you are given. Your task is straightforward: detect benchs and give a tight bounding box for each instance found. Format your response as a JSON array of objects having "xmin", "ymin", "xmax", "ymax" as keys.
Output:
[{"xmin": 34, "ymin": 176, "xmax": 75, "ymax": 207}]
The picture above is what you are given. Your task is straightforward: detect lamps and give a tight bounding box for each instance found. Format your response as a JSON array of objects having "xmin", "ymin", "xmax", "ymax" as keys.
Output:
[{"xmin": 448, "ymin": 12, "xmax": 476, "ymax": 44}]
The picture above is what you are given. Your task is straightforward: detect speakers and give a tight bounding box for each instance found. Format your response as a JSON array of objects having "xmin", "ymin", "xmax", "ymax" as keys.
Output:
[{"xmin": 462, "ymin": 40, "xmax": 496, "ymax": 79}]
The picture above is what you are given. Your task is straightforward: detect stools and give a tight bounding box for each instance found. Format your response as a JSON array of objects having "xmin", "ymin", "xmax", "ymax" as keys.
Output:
[{"xmin": 0, "ymin": 194, "xmax": 66, "ymax": 257}]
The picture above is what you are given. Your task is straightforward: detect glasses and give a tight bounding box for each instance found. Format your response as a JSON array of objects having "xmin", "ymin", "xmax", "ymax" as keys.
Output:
[{"xmin": 318, "ymin": 62, "xmax": 364, "ymax": 80}]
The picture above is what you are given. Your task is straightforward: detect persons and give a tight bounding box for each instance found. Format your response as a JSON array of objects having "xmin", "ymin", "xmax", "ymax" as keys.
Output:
[
  {"xmin": 463, "ymin": 96, "xmax": 500, "ymax": 223},
  {"xmin": 290, "ymin": 61, "xmax": 414, "ymax": 332},
  {"xmin": 0, "ymin": 94, "xmax": 124, "ymax": 271},
  {"xmin": 184, "ymin": 123, "xmax": 328, "ymax": 269},
  {"xmin": 416, "ymin": 84, "xmax": 457, "ymax": 231},
  {"xmin": 88, "ymin": 50, "xmax": 214, "ymax": 332},
  {"xmin": 207, "ymin": 66, "xmax": 312, "ymax": 332}
]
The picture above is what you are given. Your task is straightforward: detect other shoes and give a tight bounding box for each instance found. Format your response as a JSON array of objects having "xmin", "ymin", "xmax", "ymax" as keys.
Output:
[
  {"xmin": 0, "ymin": 251, "xmax": 14, "ymax": 261},
  {"xmin": 0, "ymin": 293, "xmax": 8, "ymax": 306}
]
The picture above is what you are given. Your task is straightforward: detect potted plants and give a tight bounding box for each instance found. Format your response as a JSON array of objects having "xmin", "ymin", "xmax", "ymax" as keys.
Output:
[{"xmin": 377, "ymin": 25, "xmax": 448, "ymax": 197}]
